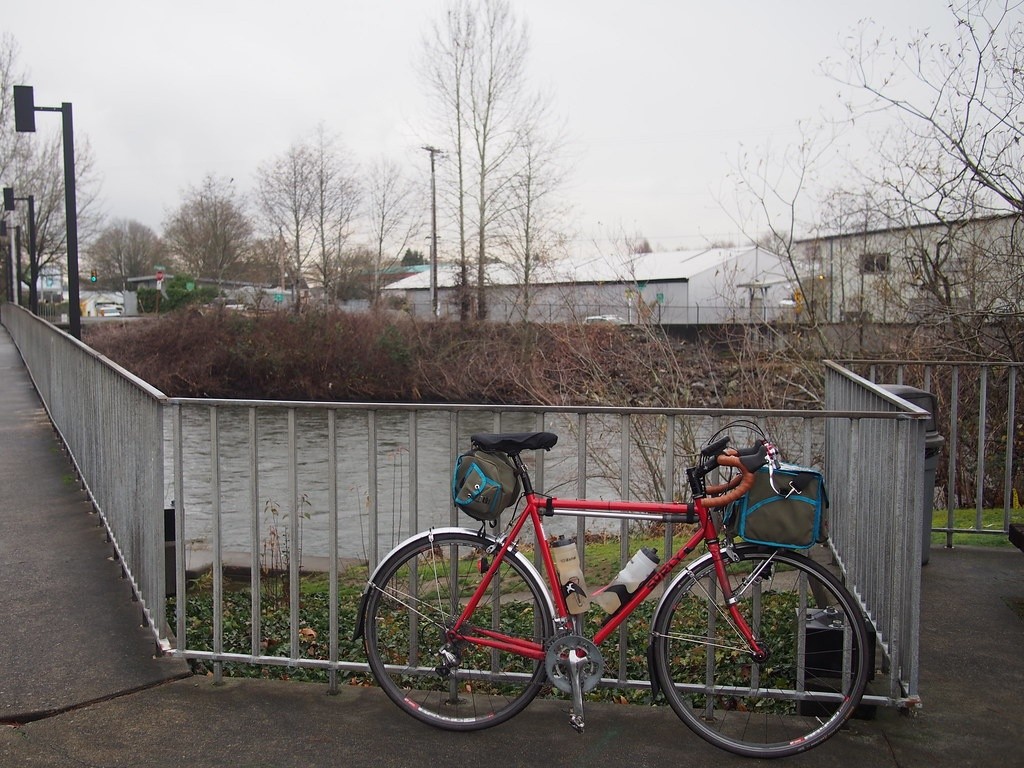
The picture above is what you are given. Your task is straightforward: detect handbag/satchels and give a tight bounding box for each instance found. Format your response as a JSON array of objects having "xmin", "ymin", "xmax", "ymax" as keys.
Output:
[
  {"xmin": 453, "ymin": 448, "xmax": 521, "ymax": 540},
  {"xmin": 723, "ymin": 462, "xmax": 830, "ymax": 549}
]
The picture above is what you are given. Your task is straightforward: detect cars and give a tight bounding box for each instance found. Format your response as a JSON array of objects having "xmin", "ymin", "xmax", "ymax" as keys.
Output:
[
  {"xmin": 583, "ymin": 314, "xmax": 633, "ymax": 325},
  {"xmin": 196, "ymin": 298, "xmax": 248, "ymax": 317}
]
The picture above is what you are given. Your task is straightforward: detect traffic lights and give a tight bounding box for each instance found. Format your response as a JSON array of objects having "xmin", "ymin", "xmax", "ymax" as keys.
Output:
[{"xmin": 92, "ymin": 270, "xmax": 97, "ymax": 282}]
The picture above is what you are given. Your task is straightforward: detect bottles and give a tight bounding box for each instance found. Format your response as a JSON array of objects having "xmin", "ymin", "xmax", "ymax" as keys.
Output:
[
  {"xmin": 553, "ymin": 534, "xmax": 591, "ymax": 615},
  {"xmin": 595, "ymin": 547, "xmax": 659, "ymax": 615}
]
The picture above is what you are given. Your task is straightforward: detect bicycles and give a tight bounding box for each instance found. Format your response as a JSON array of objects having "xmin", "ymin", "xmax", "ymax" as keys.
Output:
[{"xmin": 355, "ymin": 419, "xmax": 877, "ymax": 759}]
